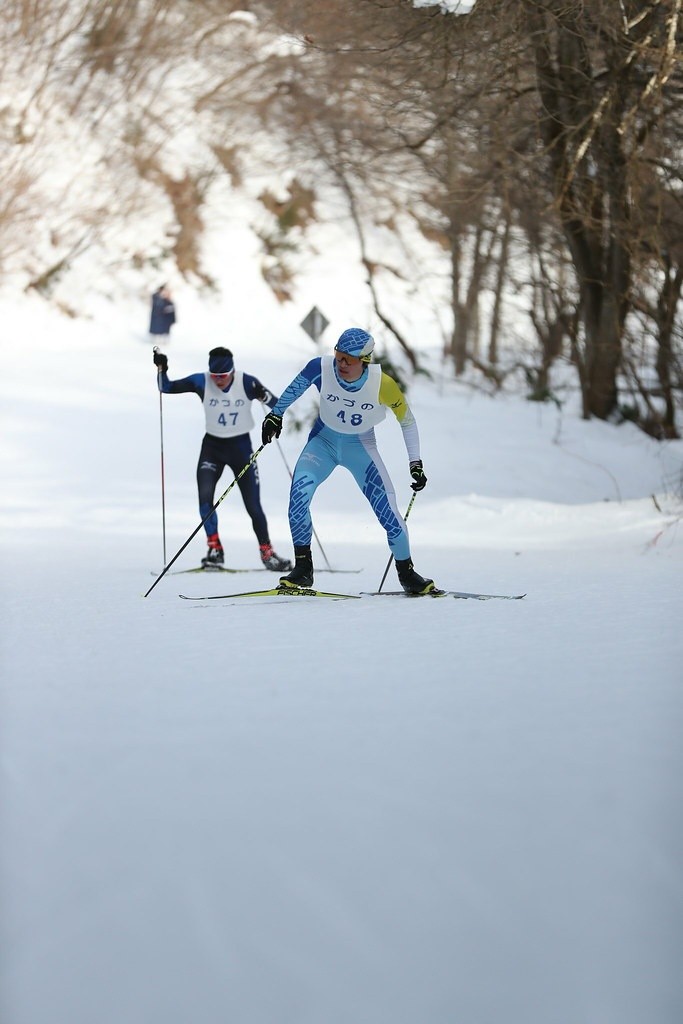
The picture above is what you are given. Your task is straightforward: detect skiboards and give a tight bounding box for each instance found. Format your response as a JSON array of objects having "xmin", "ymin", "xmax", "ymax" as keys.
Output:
[
  {"xmin": 152, "ymin": 565, "xmax": 360, "ymax": 575},
  {"xmin": 178, "ymin": 585, "xmax": 529, "ymax": 602}
]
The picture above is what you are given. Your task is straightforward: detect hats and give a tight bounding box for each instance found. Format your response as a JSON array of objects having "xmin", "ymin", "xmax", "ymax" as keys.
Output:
[
  {"xmin": 336, "ymin": 328, "xmax": 375, "ymax": 355},
  {"xmin": 208, "ymin": 347, "xmax": 233, "ymax": 372}
]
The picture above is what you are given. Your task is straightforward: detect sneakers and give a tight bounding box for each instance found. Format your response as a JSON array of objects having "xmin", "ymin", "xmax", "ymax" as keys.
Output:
[
  {"xmin": 259, "ymin": 545, "xmax": 292, "ymax": 571},
  {"xmin": 202, "ymin": 538, "xmax": 224, "ymax": 564}
]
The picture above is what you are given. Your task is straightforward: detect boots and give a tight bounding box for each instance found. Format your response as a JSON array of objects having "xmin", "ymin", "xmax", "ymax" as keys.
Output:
[
  {"xmin": 394, "ymin": 556, "xmax": 434, "ymax": 595},
  {"xmin": 279, "ymin": 545, "xmax": 314, "ymax": 589}
]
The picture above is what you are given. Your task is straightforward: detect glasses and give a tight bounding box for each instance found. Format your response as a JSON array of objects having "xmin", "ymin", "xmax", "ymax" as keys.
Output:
[
  {"xmin": 208, "ymin": 369, "xmax": 234, "ymax": 380},
  {"xmin": 334, "ymin": 344, "xmax": 365, "ymax": 366}
]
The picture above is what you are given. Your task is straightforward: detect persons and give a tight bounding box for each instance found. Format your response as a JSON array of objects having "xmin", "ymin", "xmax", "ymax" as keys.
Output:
[
  {"xmin": 261, "ymin": 328, "xmax": 434, "ymax": 595},
  {"xmin": 154, "ymin": 347, "xmax": 291, "ymax": 572},
  {"xmin": 149, "ymin": 284, "xmax": 176, "ymax": 345}
]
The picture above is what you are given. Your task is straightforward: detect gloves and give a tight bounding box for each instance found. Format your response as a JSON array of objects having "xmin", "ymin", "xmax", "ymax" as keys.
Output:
[
  {"xmin": 262, "ymin": 413, "xmax": 283, "ymax": 445},
  {"xmin": 409, "ymin": 461, "xmax": 428, "ymax": 491},
  {"xmin": 154, "ymin": 354, "xmax": 168, "ymax": 372}
]
